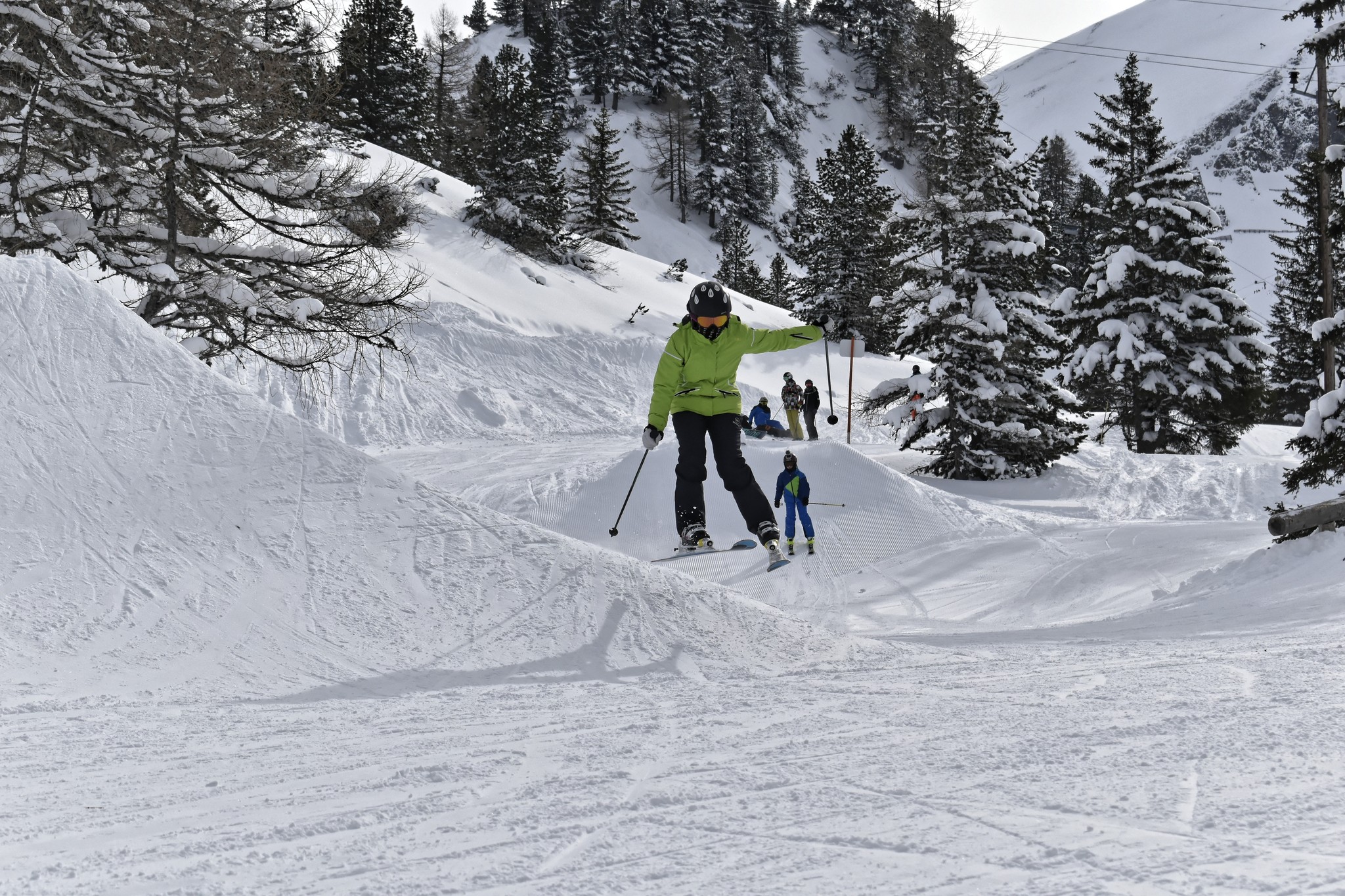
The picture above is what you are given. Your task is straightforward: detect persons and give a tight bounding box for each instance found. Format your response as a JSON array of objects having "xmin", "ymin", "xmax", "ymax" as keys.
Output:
[
  {"xmin": 910, "ymin": 365, "xmax": 924, "ymax": 421},
  {"xmin": 800, "ymin": 380, "xmax": 820, "ymax": 441},
  {"xmin": 782, "ymin": 372, "xmax": 804, "ymax": 441},
  {"xmin": 644, "ymin": 281, "xmax": 834, "ymax": 551},
  {"xmin": 775, "ymin": 450, "xmax": 816, "ymax": 546},
  {"xmin": 749, "ymin": 397, "xmax": 785, "ymax": 430}
]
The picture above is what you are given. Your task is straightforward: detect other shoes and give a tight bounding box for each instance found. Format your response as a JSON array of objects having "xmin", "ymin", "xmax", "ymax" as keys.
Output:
[
  {"xmin": 792, "ymin": 439, "xmax": 797, "ymax": 441},
  {"xmin": 806, "ymin": 437, "xmax": 818, "ymax": 441},
  {"xmin": 797, "ymin": 438, "xmax": 804, "ymax": 441}
]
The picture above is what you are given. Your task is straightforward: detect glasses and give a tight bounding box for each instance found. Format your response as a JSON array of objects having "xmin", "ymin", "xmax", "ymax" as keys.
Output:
[
  {"xmin": 805, "ymin": 383, "xmax": 811, "ymax": 387},
  {"xmin": 786, "ymin": 464, "xmax": 794, "ymax": 467},
  {"xmin": 696, "ymin": 314, "xmax": 728, "ymax": 328},
  {"xmin": 783, "ymin": 377, "xmax": 788, "ymax": 380},
  {"xmin": 761, "ymin": 401, "xmax": 767, "ymax": 405}
]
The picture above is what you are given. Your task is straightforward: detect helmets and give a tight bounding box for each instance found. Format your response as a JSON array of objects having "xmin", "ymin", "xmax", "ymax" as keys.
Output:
[
  {"xmin": 687, "ymin": 281, "xmax": 732, "ymax": 318},
  {"xmin": 805, "ymin": 380, "xmax": 813, "ymax": 389},
  {"xmin": 759, "ymin": 397, "xmax": 768, "ymax": 406},
  {"xmin": 912, "ymin": 365, "xmax": 920, "ymax": 373},
  {"xmin": 783, "ymin": 451, "xmax": 797, "ymax": 465},
  {"xmin": 783, "ymin": 372, "xmax": 793, "ymax": 381}
]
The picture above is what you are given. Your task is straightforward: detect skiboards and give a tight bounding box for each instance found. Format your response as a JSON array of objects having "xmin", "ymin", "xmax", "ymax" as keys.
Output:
[
  {"xmin": 788, "ymin": 542, "xmax": 814, "ymax": 554},
  {"xmin": 650, "ymin": 540, "xmax": 790, "ymax": 573}
]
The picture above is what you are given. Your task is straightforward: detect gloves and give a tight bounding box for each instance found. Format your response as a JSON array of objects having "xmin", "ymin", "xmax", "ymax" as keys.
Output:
[
  {"xmin": 749, "ymin": 422, "xmax": 753, "ymax": 426},
  {"xmin": 795, "ymin": 403, "xmax": 801, "ymax": 409},
  {"xmin": 641, "ymin": 424, "xmax": 664, "ymax": 449},
  {"xmin": 783, "ymin": 397, "xmax": 785, "ymax": 401},
  {"xmin": 774, "ymin": 501, "xmax": 780, "ymax": 508},
  {"xmin": 813, "ymin": 408, "xmax": 817, "ymax": 414},
  {"xmin": 802, "ymin": 499, "xmax": 809, "ymax": 506},
  {"xmin": 800, "ymin": 405, "xmax": 804, "ymax": 412},
  {"xmin": 810, "ymin": 315, "xmax": 834, "ymax": 334}
]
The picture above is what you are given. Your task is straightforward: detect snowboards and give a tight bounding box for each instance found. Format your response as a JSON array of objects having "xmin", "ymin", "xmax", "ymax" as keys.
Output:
[
  {"xmin": 742, "ymin": 429, "xmax": 767, "ymax": 439},
  {"xmin": 756, "ymin": 424, "xmax": 793, "ymax": 438}
]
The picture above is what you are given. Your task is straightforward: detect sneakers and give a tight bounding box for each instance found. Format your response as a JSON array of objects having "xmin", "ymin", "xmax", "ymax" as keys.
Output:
[
  {"xmin": 756, "ymin": 521, "xmax": 780, "ymax": 547},
  {"xmin": 786, "ymin": 537, "xmax": 794, "ymax": 545},
  {"xmin": 807, "ymin": 537, "xmax": 814, "ymax": 544},
  {"xmin": 681, "ymin": 522, "xmax": 713, "ymax": 550}
]
